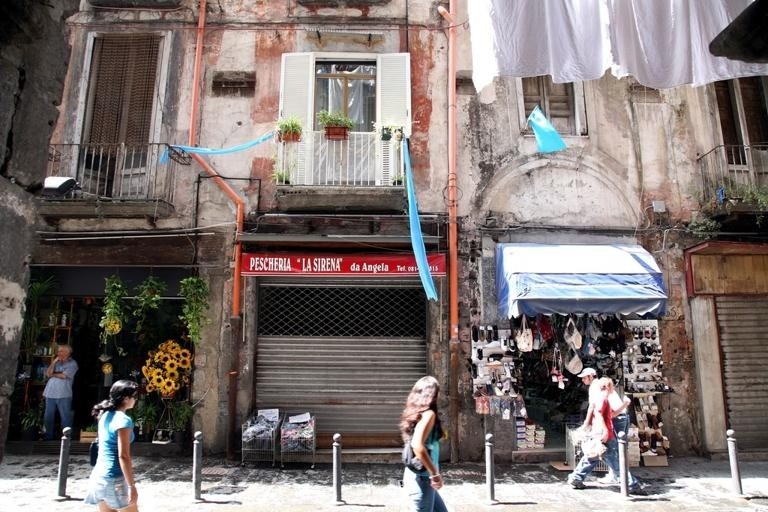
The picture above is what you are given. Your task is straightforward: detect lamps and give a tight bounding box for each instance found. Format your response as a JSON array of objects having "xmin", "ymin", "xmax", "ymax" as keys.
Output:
[{"xmin": 39, "ymin": 176, "xmax": 77, "ymax": 199}]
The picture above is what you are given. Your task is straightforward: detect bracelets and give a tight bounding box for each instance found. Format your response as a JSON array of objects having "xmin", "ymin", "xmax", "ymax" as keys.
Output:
[
  {"xmin": 429, "ymin": 473, "xmax": 440, "ymax": 480},
  {"xmin": 128, "ymin": 482, "xmax": 135, "ymax": 489}
]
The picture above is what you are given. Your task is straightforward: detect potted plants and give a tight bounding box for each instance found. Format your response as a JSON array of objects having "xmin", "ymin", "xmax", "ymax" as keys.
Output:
[
  {"xmin": 275, "ymin": 119, "xmax": 302, "ymax": 144},
  {"xmin": 314, "ymin": 109, "xmax": 352, "ymax": 141}
]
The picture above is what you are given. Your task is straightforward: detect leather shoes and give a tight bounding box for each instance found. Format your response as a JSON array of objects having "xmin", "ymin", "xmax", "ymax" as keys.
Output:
[{"xmin": 624, "ymin": 341, "xmax": 675, "ymax": 394}]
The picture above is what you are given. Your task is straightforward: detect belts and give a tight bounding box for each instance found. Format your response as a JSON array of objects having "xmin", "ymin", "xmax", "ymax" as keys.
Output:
[{"xmin": 617, "ymin": 413, "xmax": 630, "ymax": 416}]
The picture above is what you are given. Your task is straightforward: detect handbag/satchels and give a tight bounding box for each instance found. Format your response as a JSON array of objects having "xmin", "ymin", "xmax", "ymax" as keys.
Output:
[
  {"xmin": 564, "ymin": 319, "xmax": 583, "ymax": 349},
  {"xmin": 515, "ymin": 314, "xmax": 533, "ymax": 352},
  {"xmin": 585, "ymin": 317, "xmax": 597, "ymax": 340},
  {"xmin": 621, "ymin": 319, "xmax": 633, "ymax": 343},
  {"xmin": 582, "ymin": 439, "xmax": 606, "ymax": 456},
  {"xmin": 402, "ymin": 441, "xmax": 431, "ymax": 473},
  {"xmin": 565, "ymin": 343, "xmax": 583, "ymax": 374},
  {"xmin": 90, "ymin": 439, "xmax": 99, "ymax": 465}
]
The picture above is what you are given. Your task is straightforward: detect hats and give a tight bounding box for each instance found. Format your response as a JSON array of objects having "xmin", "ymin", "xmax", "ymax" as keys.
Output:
[{"xmin": 577, "ymin": 368, "xmax": 597, "ymax": 377}]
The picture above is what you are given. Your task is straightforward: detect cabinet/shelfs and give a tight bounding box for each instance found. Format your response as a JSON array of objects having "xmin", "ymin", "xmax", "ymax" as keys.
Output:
[{"xmin": 23, "ymin": 297, "xmax": 74, "ymax": 403}]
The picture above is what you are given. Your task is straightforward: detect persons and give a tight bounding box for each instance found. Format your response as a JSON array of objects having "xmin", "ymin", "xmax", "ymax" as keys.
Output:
[
  {"xmin": 568, "ymin": 376, "xmax": 649, "ymax": 495},
  {"xmin": 85, "ymin": 379, "xmax": 140, "ymax": 512},
  {"xmin": 399, "ymin": 375, "xmax": 449, "ymax": 511},
  {"xmin": 39, "ymin": 344, "xmax": 79, "ymax": 441},
  {"xmin": 577, "ymin": 368, "xmax": 629, "ymax": 485}
]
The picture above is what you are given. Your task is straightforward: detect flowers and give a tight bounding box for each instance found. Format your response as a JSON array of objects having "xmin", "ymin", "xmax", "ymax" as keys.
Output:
[{"xmin": 141, "ymin": 338, "xmax": 196, "ymax": 399}]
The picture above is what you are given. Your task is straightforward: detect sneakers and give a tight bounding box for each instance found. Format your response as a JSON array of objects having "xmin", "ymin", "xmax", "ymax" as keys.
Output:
[
  {"xmin": 596, "ymin": 474, "xmax": 618, "ymax": 484},
  {"xmin": 472, "ymin": 326, "xmax": 522, "ymax": 399},
  {"xmin": 569, "ymin": 481, "xmax": 587, "ymax": 488},
  {"xmin": 628, "ymin": 485, "xmax": 647, "ymax": 495}
]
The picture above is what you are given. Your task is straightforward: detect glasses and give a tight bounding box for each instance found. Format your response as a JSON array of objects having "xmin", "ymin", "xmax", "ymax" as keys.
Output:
[{"xmin": 124, "ymin": 394, "xmax": 138, "ymax": 400}]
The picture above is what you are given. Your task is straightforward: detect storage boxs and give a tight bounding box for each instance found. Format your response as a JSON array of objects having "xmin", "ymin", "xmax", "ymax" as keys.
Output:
[{"xmin": 628, "ymin": 441, "xmax": 642, "ymax": 467}]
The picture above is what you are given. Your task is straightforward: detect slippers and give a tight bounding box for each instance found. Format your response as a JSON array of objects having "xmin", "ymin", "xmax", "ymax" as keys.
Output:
[
  {"xmin": 39, "ymin": 435, "xmax": 52, "ymax": 440},
  {"xmin": 634, "ymin": 396, "xmax": 665, "ymax": 455}
]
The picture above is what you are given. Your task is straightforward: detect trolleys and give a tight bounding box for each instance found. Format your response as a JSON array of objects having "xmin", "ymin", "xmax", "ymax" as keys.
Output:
[
  {"xmin": 238, "ymin": 408, "xmax": 277, "ymax": 471},
  {"xmin": 277, "ymin": 409, "xmax": 318, "ymax": 471},
  {"xmin": 562, "ymin": 420, "xmax": 612, "ymax": 473}
]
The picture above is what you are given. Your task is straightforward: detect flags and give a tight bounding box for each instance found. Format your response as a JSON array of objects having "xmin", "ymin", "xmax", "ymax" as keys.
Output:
[{"xmin": 526, "ymin": 107, "xmax": 567, "ymax": 154}]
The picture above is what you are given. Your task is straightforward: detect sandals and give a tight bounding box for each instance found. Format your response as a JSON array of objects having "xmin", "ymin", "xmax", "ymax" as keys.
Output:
[{"xmin": 634, "ymin": 326, "xmax": 659, "ymax": 340}]
